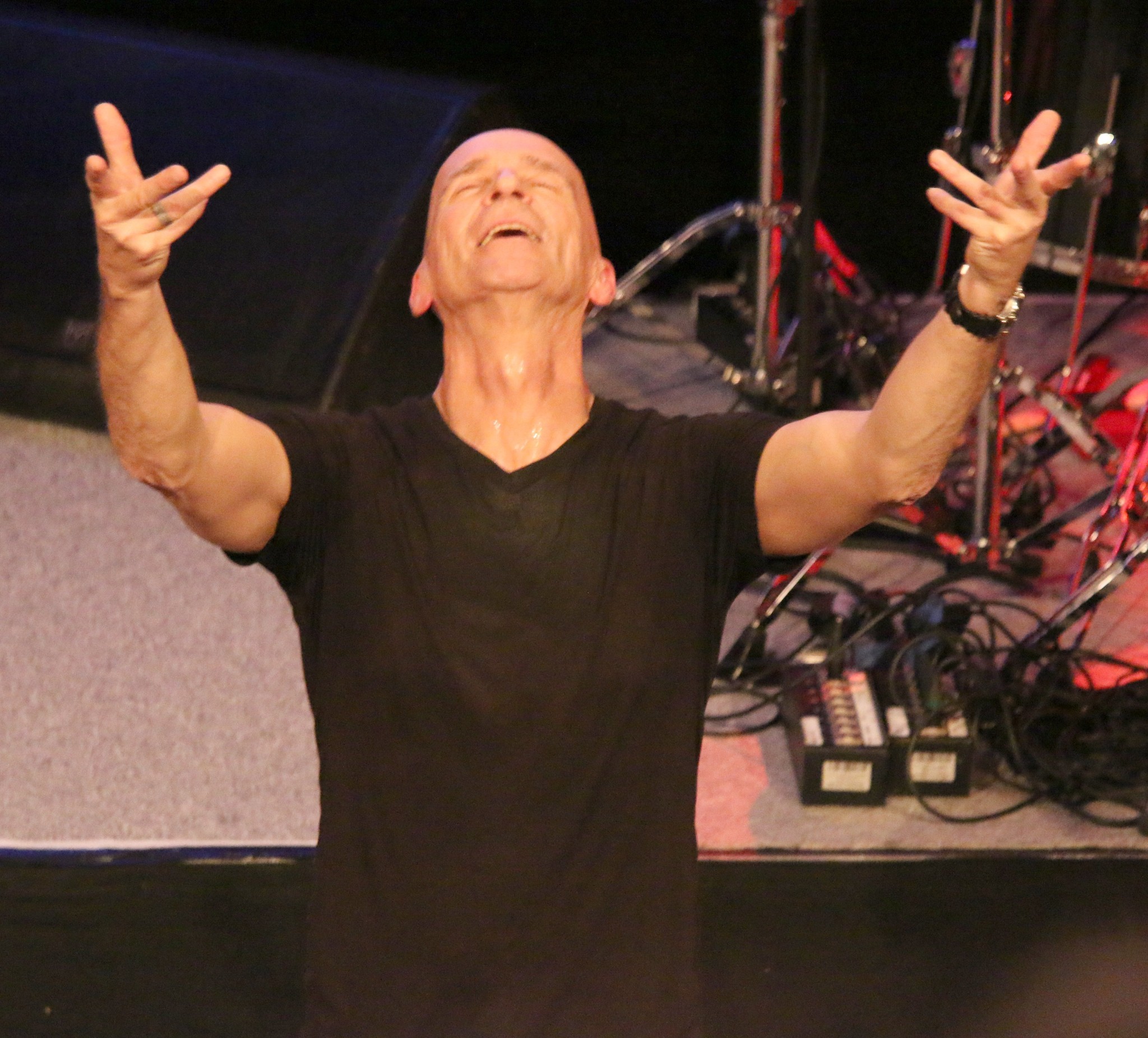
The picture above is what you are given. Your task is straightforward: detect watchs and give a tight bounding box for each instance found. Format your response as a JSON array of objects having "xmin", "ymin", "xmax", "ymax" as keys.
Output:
[{"xmin": 942, "ymin": 261, "xmax": 1026, "ymax": 340}]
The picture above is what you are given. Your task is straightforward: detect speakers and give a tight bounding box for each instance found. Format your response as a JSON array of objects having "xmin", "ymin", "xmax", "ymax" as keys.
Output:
[{"xmin": 1, "ymin": 15, "xmax": 528, "ymax": 437}]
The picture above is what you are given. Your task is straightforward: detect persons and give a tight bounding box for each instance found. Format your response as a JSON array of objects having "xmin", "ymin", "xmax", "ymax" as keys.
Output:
[{"xmin": 84, "ymin": 98, "xmax": 1093, "ymax": 1038}]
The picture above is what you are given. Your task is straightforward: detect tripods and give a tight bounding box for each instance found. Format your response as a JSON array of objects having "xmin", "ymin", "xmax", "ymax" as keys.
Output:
[{"xmin": 582, "ymin": 0, "xmax": 1147, "ymax": 683}]
